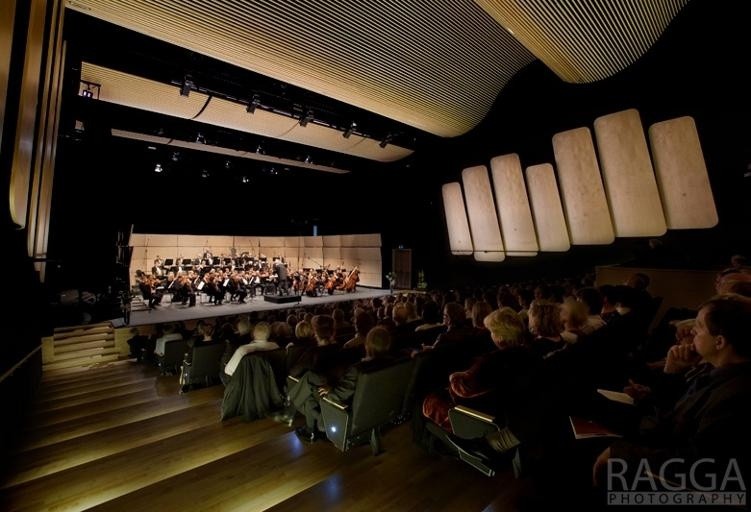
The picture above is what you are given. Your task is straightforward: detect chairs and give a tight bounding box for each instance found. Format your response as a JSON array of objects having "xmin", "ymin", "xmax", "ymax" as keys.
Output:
[
  {"xmin": 162, "ymin": 255, "xmax": 268, "ymax": 276},
  {"xmin": 144, "ymin": 300, "xmax": 657, "ymax": 479}
]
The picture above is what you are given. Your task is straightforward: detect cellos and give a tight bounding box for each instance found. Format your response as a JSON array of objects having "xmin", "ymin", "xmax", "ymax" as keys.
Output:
[{"xmin": 306, "ymin": 264, "xmax": 359, "ymax": 295}]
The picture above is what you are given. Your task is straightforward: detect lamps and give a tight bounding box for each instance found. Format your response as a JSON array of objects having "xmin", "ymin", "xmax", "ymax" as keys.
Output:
[{"xmin": 178, "ymin": 78, "xmax": 393, "ymax": 150}]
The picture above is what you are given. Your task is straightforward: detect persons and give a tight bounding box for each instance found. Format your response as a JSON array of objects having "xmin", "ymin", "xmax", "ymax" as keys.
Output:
[{"xmin": 129, "ymin": 251, "xmax": 749, "ymax": 512}]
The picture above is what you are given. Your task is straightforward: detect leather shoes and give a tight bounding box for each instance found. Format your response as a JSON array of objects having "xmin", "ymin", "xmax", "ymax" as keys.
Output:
[
  {"xmin": 295, "ymin": 427, "xmax": 314, "ymax": 441},
  {"xmin": 274, "ymin": 415, "xmax": 293, "ymax": 427}
]
